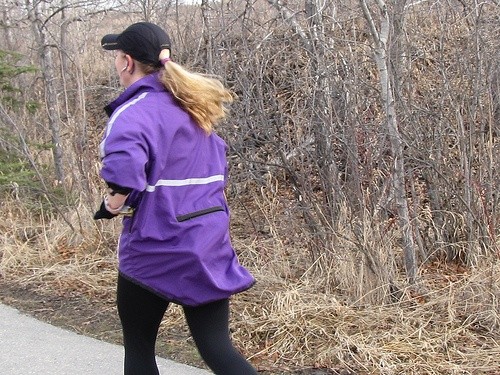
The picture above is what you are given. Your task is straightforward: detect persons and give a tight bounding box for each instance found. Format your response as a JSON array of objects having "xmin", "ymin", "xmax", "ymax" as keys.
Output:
[{"xmin": 94, "ymin": 23, "xmax": 261, "ymax": 375}]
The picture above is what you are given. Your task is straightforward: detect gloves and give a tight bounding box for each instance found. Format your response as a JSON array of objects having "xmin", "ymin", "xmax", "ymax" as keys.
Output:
[{"xmin": 92, "ymin": 197, "xmax": 124, "ymax": 220}]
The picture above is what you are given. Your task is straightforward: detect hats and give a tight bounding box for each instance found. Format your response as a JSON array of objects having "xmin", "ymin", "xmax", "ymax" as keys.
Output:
[{"xmin": 100, "ymin": 21, "xmax": 172, "ymax": 67}]
